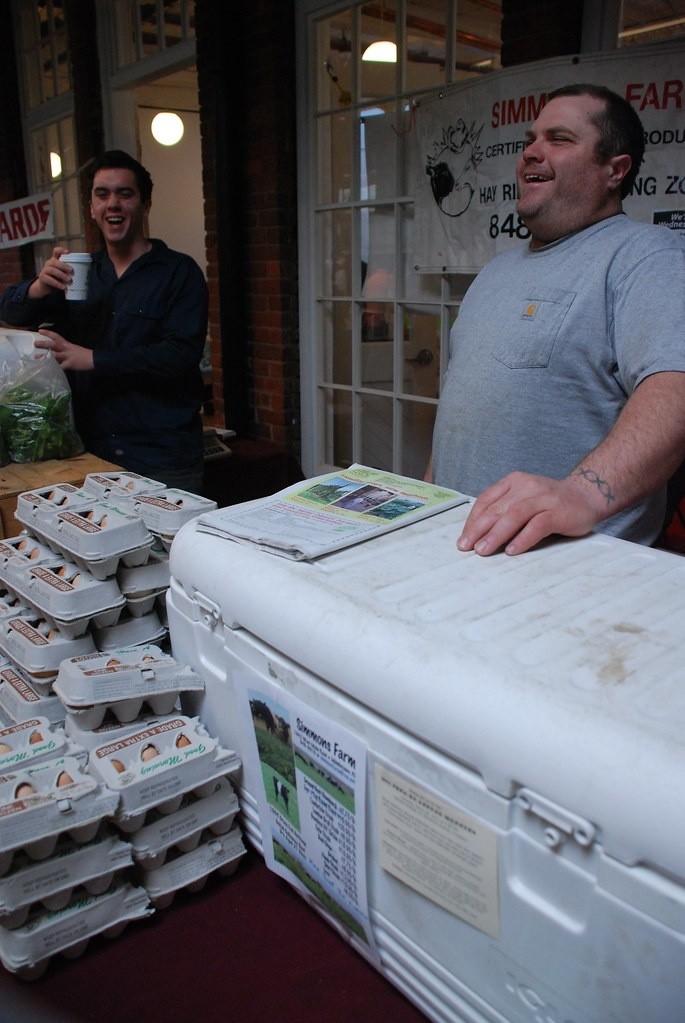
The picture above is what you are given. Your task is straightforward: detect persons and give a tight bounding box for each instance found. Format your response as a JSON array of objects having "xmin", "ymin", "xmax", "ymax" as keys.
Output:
[
  {"xmin": 422, "ymin": 84, "xmax": 685, "ymax": 556},
  {"xmin": 1, "ymin": 149, "xmax": 209, "ymax": 493}
]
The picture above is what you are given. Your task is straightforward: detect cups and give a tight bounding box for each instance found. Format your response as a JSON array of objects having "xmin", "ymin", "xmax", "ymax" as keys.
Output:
[{"xmin": 59, "ymin": 252, "xmax": 92, "ymax": 300}]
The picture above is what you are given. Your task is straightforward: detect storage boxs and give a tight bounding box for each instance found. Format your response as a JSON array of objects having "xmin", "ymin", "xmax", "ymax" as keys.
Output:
[
  {"xmin": 165, "ymin": 476, "xmax": 684, "ymax": 1023},
  {"xmin": 0, "ymin": 451, "xmax": 126, "ymax": 539}
]
